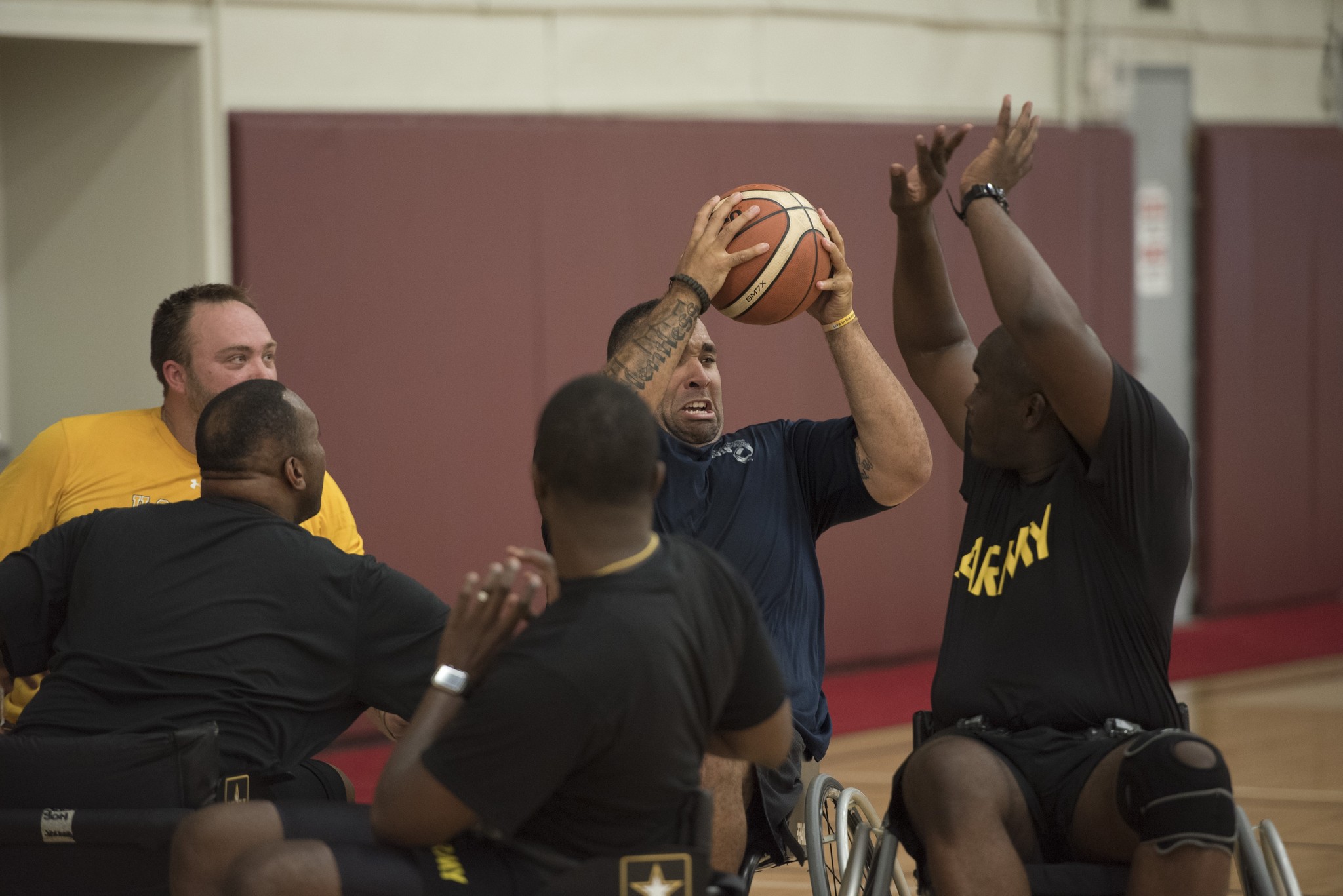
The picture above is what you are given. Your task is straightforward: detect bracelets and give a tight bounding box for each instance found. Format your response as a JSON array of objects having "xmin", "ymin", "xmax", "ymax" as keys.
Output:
[
  {"xmin": 667, "ymin": 273, "xmax": 711, "ymax": 314},
  {"xmin": 821, "ymin": 308, "xmax": 856, "ymax": 333}
]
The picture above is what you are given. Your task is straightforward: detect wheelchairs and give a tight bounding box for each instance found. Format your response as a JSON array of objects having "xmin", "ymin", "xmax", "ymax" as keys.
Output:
[
  {"xmin": 689, "ymin": 771, "xmax": 897, "ymax": 896},
  {"xmin": 835, "ymin": 707, "xmax": 1304, "ymax": 896}
]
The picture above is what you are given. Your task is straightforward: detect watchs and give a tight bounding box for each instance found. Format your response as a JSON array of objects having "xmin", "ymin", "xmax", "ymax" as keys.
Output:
[
  {"xmin": 946, "ymin": 183, "xmax": 1011, "ymax": 227},
  {"xmin": 429, "ymin": 662, "xmax": 476, "ymax": 700}
]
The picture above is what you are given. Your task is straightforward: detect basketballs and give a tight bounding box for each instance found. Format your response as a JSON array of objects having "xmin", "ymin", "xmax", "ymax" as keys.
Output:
[{"xmin": 703, "ymin": 184, "xmax": 832, "ymax": 325}]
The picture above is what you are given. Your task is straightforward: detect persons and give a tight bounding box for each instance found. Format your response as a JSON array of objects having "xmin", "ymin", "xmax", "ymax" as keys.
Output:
[
  {"xmin": 0, "ymin": 377, "xmax": 455, "ymax": 896},
  {"xmin": 538, "ymin": 189, "xmax": 934, "ymax": 896},
  {"xmin": 166, "ymin": 374, "xmax": 798, "ymax": 893},
  {"xmin": 0, "ymin": 287, "xmax": 371, "ymax": 738},
  {"xmin": 888, "ymin": 94, "xmax": 1237, "ymax": 895}
]
